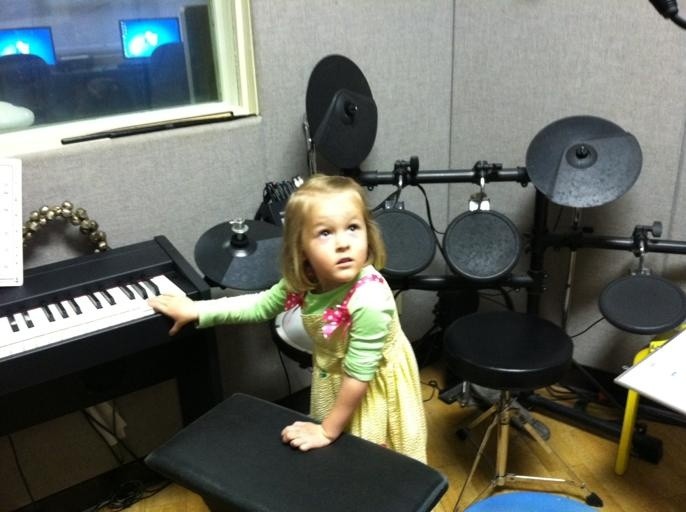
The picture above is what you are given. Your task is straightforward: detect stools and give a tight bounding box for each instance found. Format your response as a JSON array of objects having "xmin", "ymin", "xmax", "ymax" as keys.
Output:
[
  {"xmin": 144, "ymin": 389, "xmax": 448, "ymax": 512},
  {"xmin": 613, "ymin": 321, "xmax": 686, "ymax": 476},
  {"xmin": 442, "ymin": 309, "xmax": 603, "ymax": 512}
]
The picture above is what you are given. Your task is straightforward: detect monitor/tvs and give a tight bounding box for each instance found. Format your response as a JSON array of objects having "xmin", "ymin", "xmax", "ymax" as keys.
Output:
[
  {"xmin": 118, "ymin": 16, "xmax": 182, "ymax": 60},
  {"xmin": 0, "ymin": 26, "xmax": 58, "ymax": 67}
]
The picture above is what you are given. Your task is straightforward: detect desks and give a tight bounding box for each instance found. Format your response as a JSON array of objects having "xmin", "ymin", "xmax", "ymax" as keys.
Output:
[{"xmin": 51, "ymin": 62, "xmax": 147, "ymax": 81}]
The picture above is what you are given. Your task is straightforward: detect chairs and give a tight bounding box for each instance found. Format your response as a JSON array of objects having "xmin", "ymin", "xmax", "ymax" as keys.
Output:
[
  {"xmin": 146, "ymin": 42, "xmax": 189, "ymax": 110},
  {"xmin": 0, "ymin": 54, "xmax": 59, "ymax": 127}
]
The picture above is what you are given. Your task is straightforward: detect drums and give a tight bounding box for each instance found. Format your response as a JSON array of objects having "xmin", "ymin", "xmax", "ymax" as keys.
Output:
[
  {"xmin": 597, "ymin": 272, "xmax": 685, "ymax": 333},
  {"xmin": 369, "ymin": 208, "xmax": 437, "ymax": 278},
  {"xmin": 444, "ymin": 210, "xmax": 522, "ymax": 281}
]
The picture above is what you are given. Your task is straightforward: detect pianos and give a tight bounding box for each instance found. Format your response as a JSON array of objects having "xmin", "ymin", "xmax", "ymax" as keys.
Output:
[{"xmin": 0, "ymin": 235, "xmax": 213, "ymax": 511}]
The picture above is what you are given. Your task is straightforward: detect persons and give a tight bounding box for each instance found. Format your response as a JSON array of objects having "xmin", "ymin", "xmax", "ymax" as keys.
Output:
[{"xmin": 146, "ymin": 176, "xmax": 430, "ymax": 467}]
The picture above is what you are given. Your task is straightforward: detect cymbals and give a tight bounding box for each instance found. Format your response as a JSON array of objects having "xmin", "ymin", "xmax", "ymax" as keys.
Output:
[
  {"xmin": 308, "ymin": 54, "xmax": 378, "ymax": 170},
  {"xmin": 194, "ymin": 218, "xmax": 286, "ymax": 292},
  {"xmin": 524, "ymin": 116, "xmax": 644, "ymax": 208}
]
